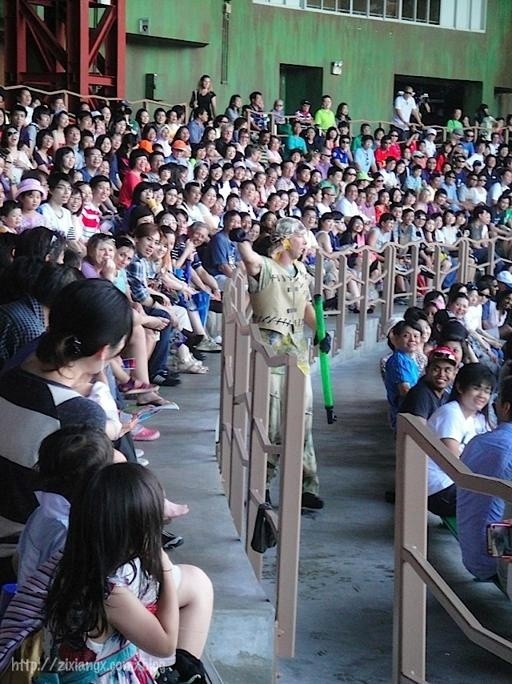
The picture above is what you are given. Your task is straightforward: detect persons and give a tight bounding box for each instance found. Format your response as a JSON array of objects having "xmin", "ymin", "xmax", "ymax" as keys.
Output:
[
  {"xmin": 456, "ymin": 376, "xmax": 512, "ymax": 583},
  {"xmin": 0, "ymin": 265, "xmax": 184, "ymax": 551},
  {"xmin": 0, "ymin": 73, "xmax": 512, "ymax": 450},
  {"xmin": 0, "ymin": 462, "xmax": 215, "ymax": 683},
  {"xmin": 425, "ymin": 362, "xmax": 496, "ymax": 516},
  {"xmin": 11, "ymin": 424, "xmax": 114, "ymax": 583},
  {"xmin": 0, "ymin": 277, "xmax": 190, "ymax": 519},
  {"xmin": 228, "ymin": 217, "xmax": 331, "ymax": 510}
]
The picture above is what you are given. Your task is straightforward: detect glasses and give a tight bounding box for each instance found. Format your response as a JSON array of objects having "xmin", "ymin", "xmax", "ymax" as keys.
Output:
[
  {"xmin": 7, "ymin": 131, "xmax": 18, "ymax": 136},
  {"xmin": 342, "ymin": 127, "xmax": 487, "ymax": 182}
]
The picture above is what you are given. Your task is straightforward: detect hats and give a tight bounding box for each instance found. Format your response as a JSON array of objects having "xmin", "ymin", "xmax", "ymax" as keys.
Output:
[
  {"xmin": 496, "ymin": 269, "xmax": 512, "ymax": 285},
  {"xmin": 91, "ymin": 111, "xmax": 104, "ymax": 119},
  {"xmin": 171, "ymin": 140, "xmax": 187, "ymax": 151},
  {"xmin": 193, "ymin": 161, "xmax": 209, "ymax": 171},
  {"xmin": 427, "ymin": 347, "xmax": 458, "ymax": 364},
  {"xmin": 14, "ymin": 178, "xmax": 47, "ymax": 202},
  {"xmin": 134, "ymin": 181, "xmax": 154, "ymax": 202}
]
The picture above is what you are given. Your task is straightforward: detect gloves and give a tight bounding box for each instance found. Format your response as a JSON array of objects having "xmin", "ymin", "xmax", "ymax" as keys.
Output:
[
  {"xmin": 314, "ymin": 330, "xmax": 331, "ymax": 354},
  {"xmin": 229, "ymin": 227, "xmax": 247, "ymax": 243}
]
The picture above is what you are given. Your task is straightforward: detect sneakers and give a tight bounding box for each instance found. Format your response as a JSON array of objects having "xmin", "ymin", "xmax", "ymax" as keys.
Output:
[
  {"xmin": 321, "ymin": 289, "xmax": 435, "ymax": 317},
  {"xmin": 118, "ymin": 336, "xmax": 225, "ymax": 552},
  {"xmin": 300, "ymin": 492, "xmax": 323, "ymax": 512}
]
